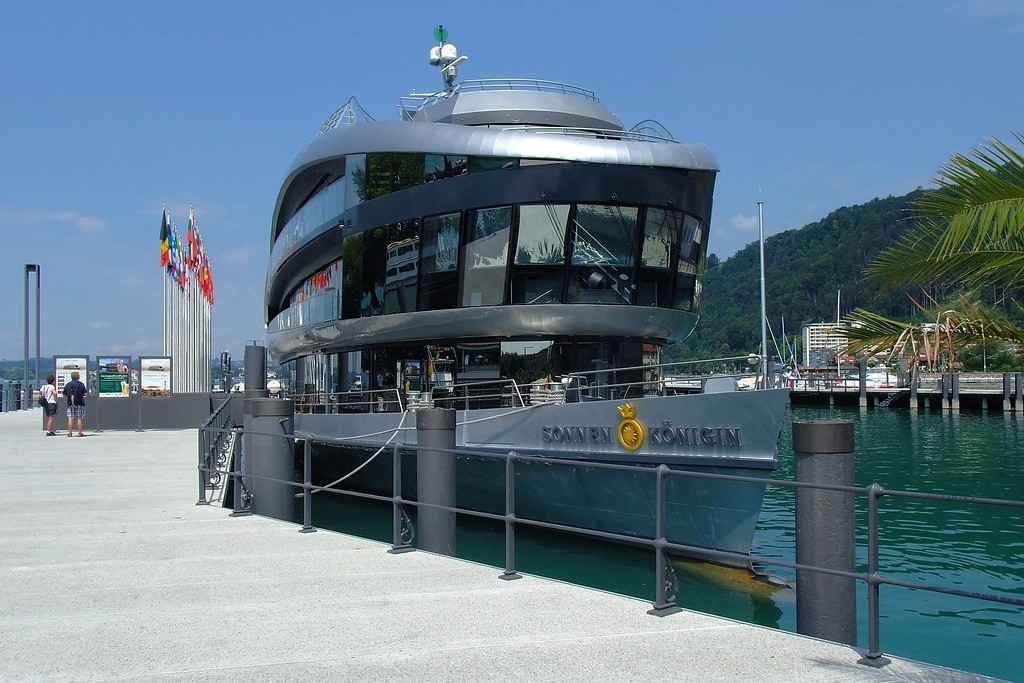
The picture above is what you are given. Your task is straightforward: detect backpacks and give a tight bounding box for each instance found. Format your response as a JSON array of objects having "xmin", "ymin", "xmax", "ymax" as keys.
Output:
[
  {"xmin": 67, "ymin": 382, "xmax": 81, "ymax": 406},
  {"xmin": 38, "ymin": 385, "xmax": 53, "ymax": 407}
]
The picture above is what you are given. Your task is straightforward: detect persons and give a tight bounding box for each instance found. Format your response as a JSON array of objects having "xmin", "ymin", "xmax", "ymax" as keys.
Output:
[
  {"xmin": 62, "ymin": 372, "xmax": 88, "ymax": 437},
  {"xmin": 37, "ymin": 375, "xmax": 58, "ymax": 436}
]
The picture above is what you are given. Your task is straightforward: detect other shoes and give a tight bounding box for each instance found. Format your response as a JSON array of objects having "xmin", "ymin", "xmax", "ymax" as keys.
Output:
[
  {"xmin": 46, "ymin": 431, "xmax": 56, "ymax": 436},
  {"xmin": 78, "ymin": 433, "xmax": 86, "ymax": 437},
  {"xmin": 67, "ymin": 433, "xmax": 72, "ymax": 437}
]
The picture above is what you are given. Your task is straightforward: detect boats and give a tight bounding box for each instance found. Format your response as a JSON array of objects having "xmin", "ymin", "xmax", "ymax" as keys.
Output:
[{"xmin": 260, "ymin": 23, "xmax": 795, "ymax": 566}]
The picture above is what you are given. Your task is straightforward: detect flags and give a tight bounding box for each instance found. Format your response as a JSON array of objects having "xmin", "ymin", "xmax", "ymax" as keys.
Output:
[
  {"xmin": 289, "ymin": 260, "xmax": 338, "ymax": 305},
  {"xmin": 159, "ymin": 205, "xmax": 214, "ymax": 306}
]
